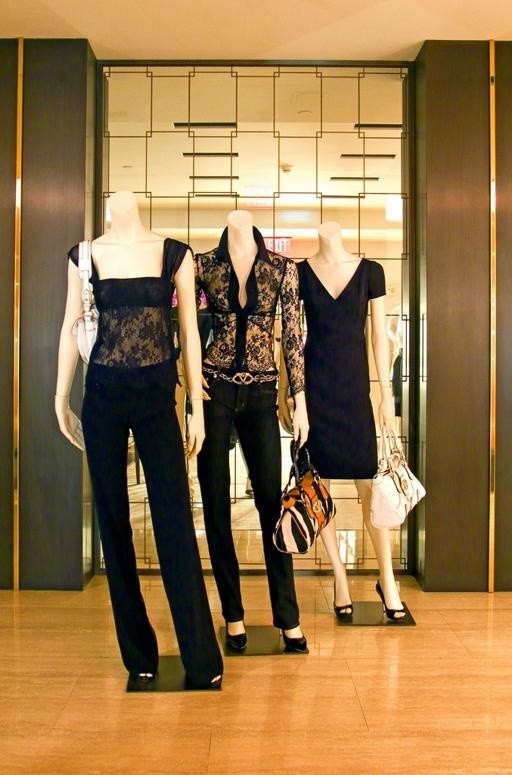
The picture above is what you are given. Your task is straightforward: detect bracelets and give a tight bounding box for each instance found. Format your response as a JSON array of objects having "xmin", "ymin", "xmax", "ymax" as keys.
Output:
[{"xmin": 55, "ymin": 395, "xmax": 70, "ymax": 401}]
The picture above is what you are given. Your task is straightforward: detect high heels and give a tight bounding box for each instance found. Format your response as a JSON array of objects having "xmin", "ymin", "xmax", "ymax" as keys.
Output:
[
  {"xmin": 226, "ymin": 620, "xmax": 247, "ymax": 652},
  {"xmin": 279, "ymin": 627, "xmax": 307, "ymax": 650},
  {"xmin": 376, "ymin": 579, "xmax": 407, "ymax": 620},
  {"xmin": 332, "ymin": 580, "xmax": 353, "ymax": 618}
]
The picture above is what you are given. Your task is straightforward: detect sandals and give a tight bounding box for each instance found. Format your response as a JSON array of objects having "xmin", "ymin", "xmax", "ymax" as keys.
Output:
[
  {"xmin": 210, "ymin": 675, "xmax": 222, "ymax": 688},
  {"xmin": 139, "ymin": 672, "xmax": 153, "ymax": 683}
]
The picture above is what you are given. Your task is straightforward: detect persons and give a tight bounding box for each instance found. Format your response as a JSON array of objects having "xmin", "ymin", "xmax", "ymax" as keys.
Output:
[
  {"xmin": 174, "ymin": 209, "xmax": 313, "ymax": 654},
  {"xmin": 277, "ymin": 218, "xmax": 406, "ymax": 624},
  {"xmin": 53, "ymin": 189, "xmax": 224, "ymax": 687}
]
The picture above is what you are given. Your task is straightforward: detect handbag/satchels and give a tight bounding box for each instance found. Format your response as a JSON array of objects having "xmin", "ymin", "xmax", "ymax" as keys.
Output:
[
  {"xmin": 273, "ymin": 468, "xmax": 337, "ymax": 554},
  {"xmin": 369, "ymin": 460, "xmax": 427, "ymax": 529},
  {"xmin": 72, "ymin": 309, "xmax": 99, "ymax": 364}
]
